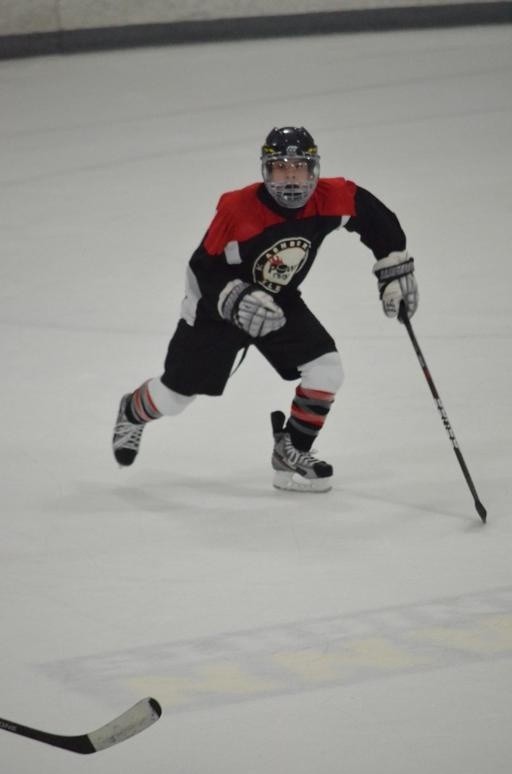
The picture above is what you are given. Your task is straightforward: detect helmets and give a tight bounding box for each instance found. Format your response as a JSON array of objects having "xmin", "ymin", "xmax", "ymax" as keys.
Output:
[{"xmin": 260, "ymin": 126, "xmax": 320, "ymax": 177}]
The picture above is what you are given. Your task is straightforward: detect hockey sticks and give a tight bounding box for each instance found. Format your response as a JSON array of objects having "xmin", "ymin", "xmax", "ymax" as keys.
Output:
[
  {"xmin": 2, "ymin": 699, "xmax": 162, "ymax": 754},
  {"xmin": 398, "ymin": 300, "xmax": 488, "ymax": 522}
]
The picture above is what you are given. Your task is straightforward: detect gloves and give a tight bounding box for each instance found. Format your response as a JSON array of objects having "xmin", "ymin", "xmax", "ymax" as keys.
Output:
[
  {"xmin": 217, "ymin": 279, "xmax": 287, "ymax": 338},
  {"xmin": 372, "ymin": 251, "xmax": 420, "ymax": 322}
]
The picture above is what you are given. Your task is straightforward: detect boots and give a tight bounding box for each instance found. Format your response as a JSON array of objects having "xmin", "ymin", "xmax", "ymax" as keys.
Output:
[
  {"xmin": 271, "ymin": 411, "xmax": 333, "ymax": 479},
  {"xmin": 113, "ymin": 393, "xmax": 145, "ymax": 465}
]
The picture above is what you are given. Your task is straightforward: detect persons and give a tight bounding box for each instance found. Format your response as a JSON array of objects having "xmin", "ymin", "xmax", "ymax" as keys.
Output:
[{"xmin": 114, "ymin": 126, "xmax": 418, "ymax": 481}]
